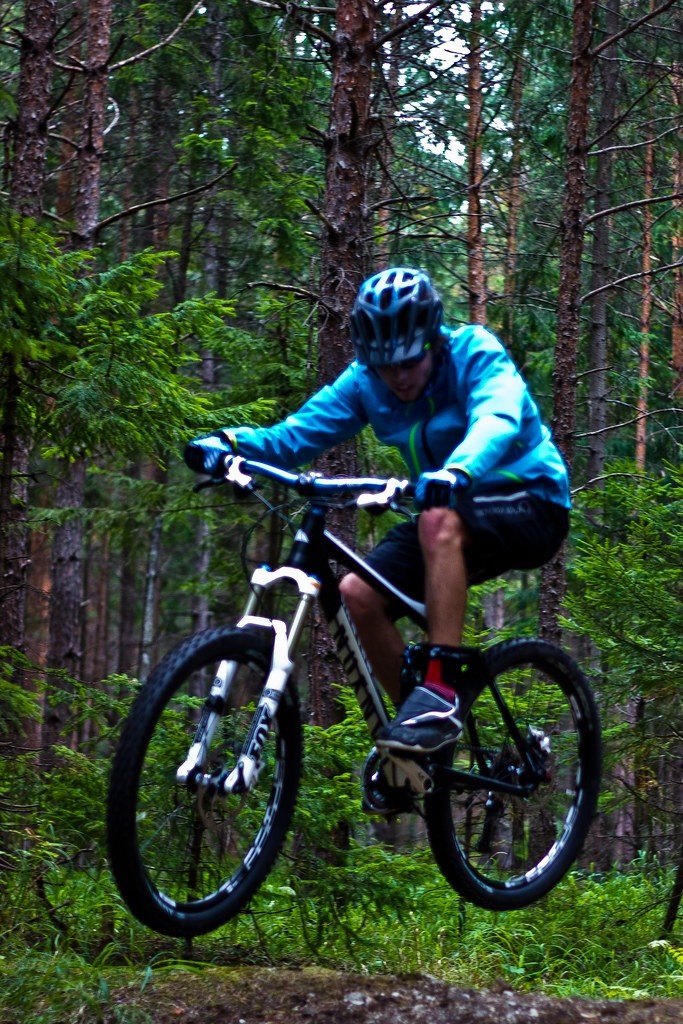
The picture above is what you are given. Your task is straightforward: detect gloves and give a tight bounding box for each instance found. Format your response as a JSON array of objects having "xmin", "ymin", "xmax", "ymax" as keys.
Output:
[
  {"xmin": 183, "ymin": 428, "xmax": 239, "ymax": 479},
  {"xmin": 413, "ymin": 469, "xmax": 469, "ymax": 514}
]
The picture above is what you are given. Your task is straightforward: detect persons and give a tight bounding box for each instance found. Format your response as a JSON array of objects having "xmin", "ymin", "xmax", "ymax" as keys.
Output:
[{"xmin": 185, "ymin": 267, "xmax": 572, "ymax": 816}]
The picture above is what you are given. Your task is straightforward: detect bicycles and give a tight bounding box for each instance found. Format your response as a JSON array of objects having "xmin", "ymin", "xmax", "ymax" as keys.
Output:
[{"xmin": 103, "ymin": 454, "xmax": 602, "ymax": 940}]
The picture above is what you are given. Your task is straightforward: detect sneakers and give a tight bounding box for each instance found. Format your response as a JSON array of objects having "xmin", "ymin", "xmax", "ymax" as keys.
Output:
[{"xmin": 374, "ymin": 683, "xmax": 466, "ymax": 755}]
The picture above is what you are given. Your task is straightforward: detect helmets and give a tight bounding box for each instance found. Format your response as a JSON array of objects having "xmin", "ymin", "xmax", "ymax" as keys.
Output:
[{"xmin": 348, "ymin": 267, "xmax": 444, "ymax": 366}]
